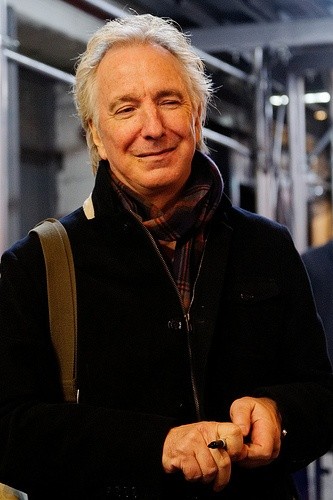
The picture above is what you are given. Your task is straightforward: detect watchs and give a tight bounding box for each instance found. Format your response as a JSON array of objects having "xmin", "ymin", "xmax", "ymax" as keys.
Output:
[{"xmin": 280, "ymin": 419, "xmax": 288, "ymax": 443}]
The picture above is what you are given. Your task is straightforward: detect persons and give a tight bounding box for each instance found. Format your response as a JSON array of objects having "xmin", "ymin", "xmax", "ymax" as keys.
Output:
[{"xmin": 0, "ymin": 8, "xmax": 333, "ymax": 500}]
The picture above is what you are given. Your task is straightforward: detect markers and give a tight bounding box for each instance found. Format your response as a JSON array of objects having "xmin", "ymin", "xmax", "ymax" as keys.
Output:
[{"xmin": 207, "ymin": 438, "xmax": 226, "ymax": 449}]
